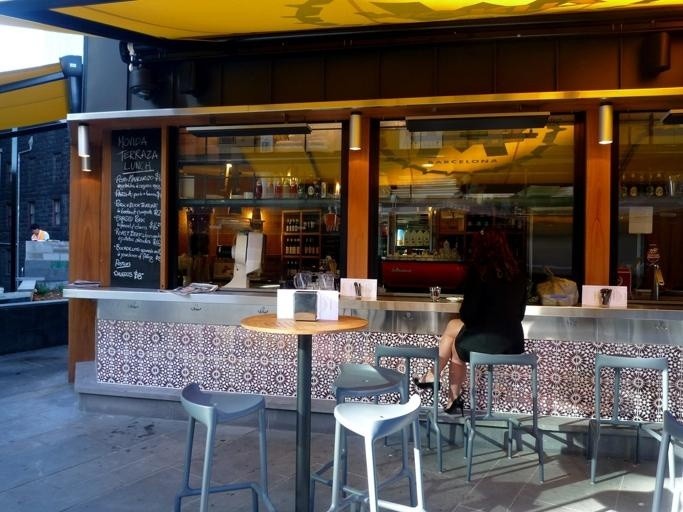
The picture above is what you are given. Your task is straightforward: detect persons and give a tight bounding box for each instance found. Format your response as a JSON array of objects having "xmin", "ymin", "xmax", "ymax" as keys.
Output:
[
  {"xmin": 409, "ymin": 221, "xmax": 523, "ymax": 417},
  {"xmin": 29, "ymin": 223, "xmax": 49, "ymax": 241}
]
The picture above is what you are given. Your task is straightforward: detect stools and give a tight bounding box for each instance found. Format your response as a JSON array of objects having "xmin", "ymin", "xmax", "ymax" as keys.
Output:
[
  {"xmin": 651, "ymin": 410, "xmax": 682, "ymax": 510},
  {"xmin": 311, "ymin": 364, "xmax": 415, "ymax": 512},
  {"xmin": 375, "ymin": 344, "xmax": 444, "ymax": 474},
  {"xmin": 173, "ymin": 382, "xmax": 274, "ymax": 512},
  {"xmin": 463, "ymin": 352, "xmax": 545, "ymax": 485},
  {"xmin": 329, "ymin": 394, "xmax": 425, "ymax": 512},
  {"xmin": 587, "ymin": 352, "xmax": 676, "ymax": 490}
]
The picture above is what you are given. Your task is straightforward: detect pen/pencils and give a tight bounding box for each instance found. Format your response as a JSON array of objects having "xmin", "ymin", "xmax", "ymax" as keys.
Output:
[
  {"xmin": 354, "ymin": 282, "xmax": 361, "ymax": 296},
  {"xmin": 600, "ymin": 288, "xmax": 613, "ymax": 306}
]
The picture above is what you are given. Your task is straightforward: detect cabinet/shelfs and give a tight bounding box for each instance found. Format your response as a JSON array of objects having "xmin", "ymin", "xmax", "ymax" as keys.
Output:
[
  {"xmin": 185, "ymin": 210, "xmax": 340, "ymax": 288},
  {"xmin": 386, "ymin": 208, "xmax": 437, "ymax": 256}
]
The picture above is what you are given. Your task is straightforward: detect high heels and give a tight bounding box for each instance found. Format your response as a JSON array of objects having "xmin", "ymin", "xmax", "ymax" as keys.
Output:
[
  {"xmin": 444, "ymin": 395, "xmax": 464, "ymax": 417},
  {"xmin": 413, "ymin": 376, "xmax": 440, "ymax": 400}
]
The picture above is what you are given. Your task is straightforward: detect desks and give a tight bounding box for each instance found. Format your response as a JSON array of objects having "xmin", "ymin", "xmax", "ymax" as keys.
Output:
[{"xmin": 239, "ymin": 305, "xmax": 369, "ymax": 512}]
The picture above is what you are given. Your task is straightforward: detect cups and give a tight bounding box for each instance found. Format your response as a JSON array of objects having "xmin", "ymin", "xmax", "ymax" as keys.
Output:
[
  {"xmin": 430, "ymin": 286, "xmax": 442, "ymax": 302},
  {"xmin": 293, "ymin": 273, "xmax": 334, "ymax": 290}
]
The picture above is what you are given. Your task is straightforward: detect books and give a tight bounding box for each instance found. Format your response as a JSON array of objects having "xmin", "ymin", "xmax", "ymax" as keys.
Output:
[
  {"xmin": 67, "ymin": 279, "xmax": 101, "ymax": 287},
  {"xmin": 160, "ymin": 282, "xmax": 218, "ymax": 296}
]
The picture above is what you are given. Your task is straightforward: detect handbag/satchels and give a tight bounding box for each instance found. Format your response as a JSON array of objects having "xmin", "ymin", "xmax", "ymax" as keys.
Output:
[{"xmin": 536, "ymin": 266, "xmax": 579, "ymax": 306}]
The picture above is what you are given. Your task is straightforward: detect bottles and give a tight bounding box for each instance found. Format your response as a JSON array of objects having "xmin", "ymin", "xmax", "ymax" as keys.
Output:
[
  {"xmin": 620, "ymin": 172, "xmax": 682, "ymax": 197},
  {"xmin": 397, "ymin": 229, "xmax": 429, "ymax": 246},
  {"xmin": 255, "ymin": 173, "xmax": 340, "ymax": 277}
]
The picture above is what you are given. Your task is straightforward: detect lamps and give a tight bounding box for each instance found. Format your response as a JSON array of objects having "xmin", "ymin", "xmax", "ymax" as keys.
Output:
[
  {"xmin": 599, "ymin": 102, "xmax": 613, "ymax": 145},
  {"xmin": 348, "ymin": 112, "xmax": 362, "ymax": 151}
]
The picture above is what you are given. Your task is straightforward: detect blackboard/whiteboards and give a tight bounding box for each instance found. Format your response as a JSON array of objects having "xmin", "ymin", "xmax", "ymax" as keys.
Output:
[{"xmin": 110, "ymin": 126, "xmax": 167, "ymax": 289}]
